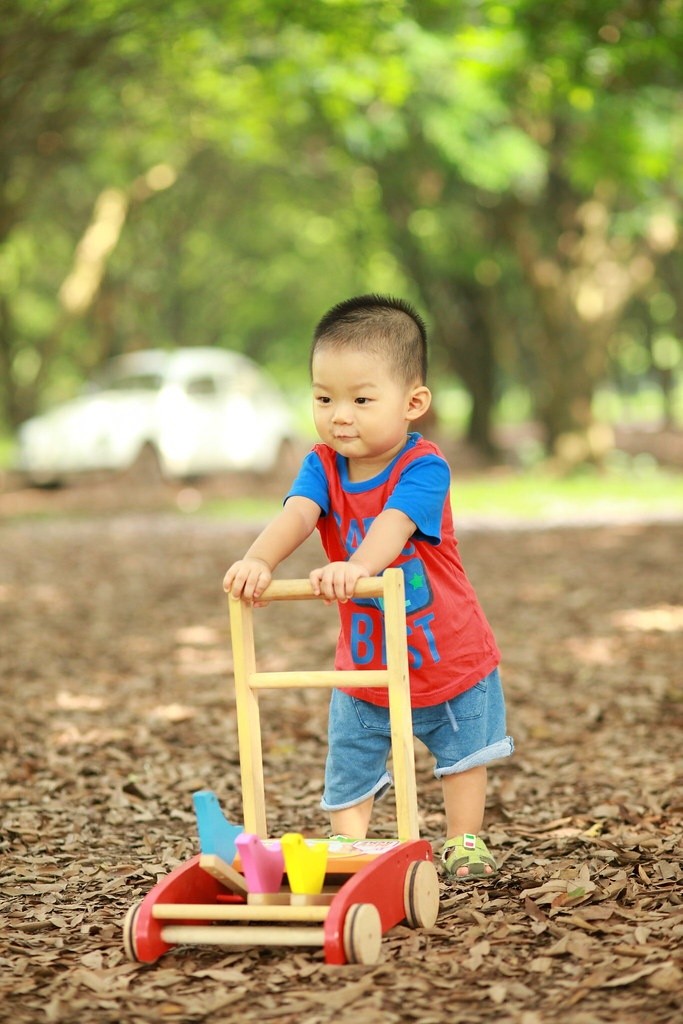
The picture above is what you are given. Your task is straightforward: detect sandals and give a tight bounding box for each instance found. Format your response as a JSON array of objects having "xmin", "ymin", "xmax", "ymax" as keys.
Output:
[{"xmin": 442, "ymin": 834, "xmax": 499, "ymax": 882}]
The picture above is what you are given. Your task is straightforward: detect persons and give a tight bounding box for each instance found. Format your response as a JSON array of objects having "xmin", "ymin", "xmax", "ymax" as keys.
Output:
[{"xmin": 223, "ymin": 293, "xmax": 514, "ymax": 881}]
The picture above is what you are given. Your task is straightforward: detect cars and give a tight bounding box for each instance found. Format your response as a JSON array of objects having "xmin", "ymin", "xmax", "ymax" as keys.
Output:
[{"xmin": 14, "ymin": 342, "xmax": 300, "ymax": 487}]
